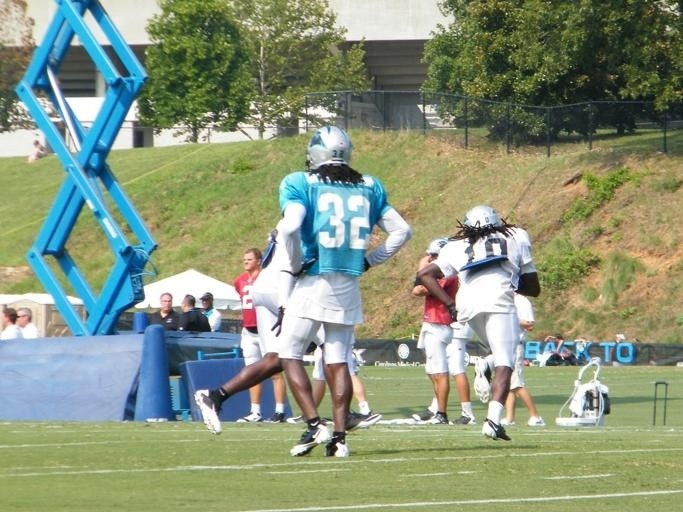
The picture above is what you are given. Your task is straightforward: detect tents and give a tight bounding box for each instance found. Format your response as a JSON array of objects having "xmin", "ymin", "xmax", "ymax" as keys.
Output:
[{"xmin": 134, "ymin": 269, "xmax": 243, "ymax": 310}]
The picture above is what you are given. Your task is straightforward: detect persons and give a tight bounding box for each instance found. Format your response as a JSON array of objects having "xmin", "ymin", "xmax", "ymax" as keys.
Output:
[
  {"xmin": 147, "ymin": 291, "xmax": 221, "ymax": 332},
  {"xmin": 234, "ymin": 248, "xmax": 287, "ymax": 423},
  {"xmin": 26, "ymin": 138, "xmax": 48, "ymax": 165},
  {"xmin": 268, "ymin": 123, "xmax": 414, "ymax": 459},
  {"xmin": 287, "ymin": 345, "xmax": 370, "ymax": 427},
  {"xmin": 534, "ymin": 332, "xmax": 632, "ymax": 368},
  {"xmin": 409, "ymin": 205, "xmax": 547, "ymax": 442},
  {"xmin": 16, "ymin": 308, "xmax": 40, "ymax": 339},
  {"xmin": 0, "ymin": 306, "xmax": 25, "ymax": 340}
]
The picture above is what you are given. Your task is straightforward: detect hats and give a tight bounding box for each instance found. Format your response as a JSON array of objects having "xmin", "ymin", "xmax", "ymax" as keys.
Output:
[{"xmin": 199, "ymin": 292, "xmax": 213, "ymax": 301}]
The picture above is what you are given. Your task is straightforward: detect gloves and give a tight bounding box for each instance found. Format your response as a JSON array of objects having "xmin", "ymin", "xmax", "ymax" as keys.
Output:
[
  {"xmin": 448, "ymin": 303, "xmax": 458, "ymax": 321},
  {"xmin": 271, "ymin": 309, "xmax": 283, "ymax": 337}
]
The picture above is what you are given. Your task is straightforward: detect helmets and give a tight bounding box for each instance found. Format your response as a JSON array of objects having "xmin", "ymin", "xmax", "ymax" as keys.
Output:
[
  {"xmin": 462, "ymin": 205, "xmax": 501, "ymax": 231},
  {"xmin": 305, "ymin": 125, "xmax": 352, "ymax": 171},
  {"xmin": 425, "ymin": 239, "xmax": 447, "ymax": 254}
]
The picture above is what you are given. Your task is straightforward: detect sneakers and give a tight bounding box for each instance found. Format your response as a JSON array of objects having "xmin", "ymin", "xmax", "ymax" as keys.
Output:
[
  {"xmin": 289, "ymin": 422, "xmax": 332, "ymax": 457},
  {"xmin": 481, "ymin": 417, "xmax": 510, "ymax": 441},
  {"xmin": 472, "ymin": 357, "xmax": 491, "ymax": 405},
  {"xmin": 325, "ymin": 435, "xmax": 348, "ymax": 457},
  {"xmin": 193, "ymin": 389, "xmax": 221, "ymax": 435}
]
